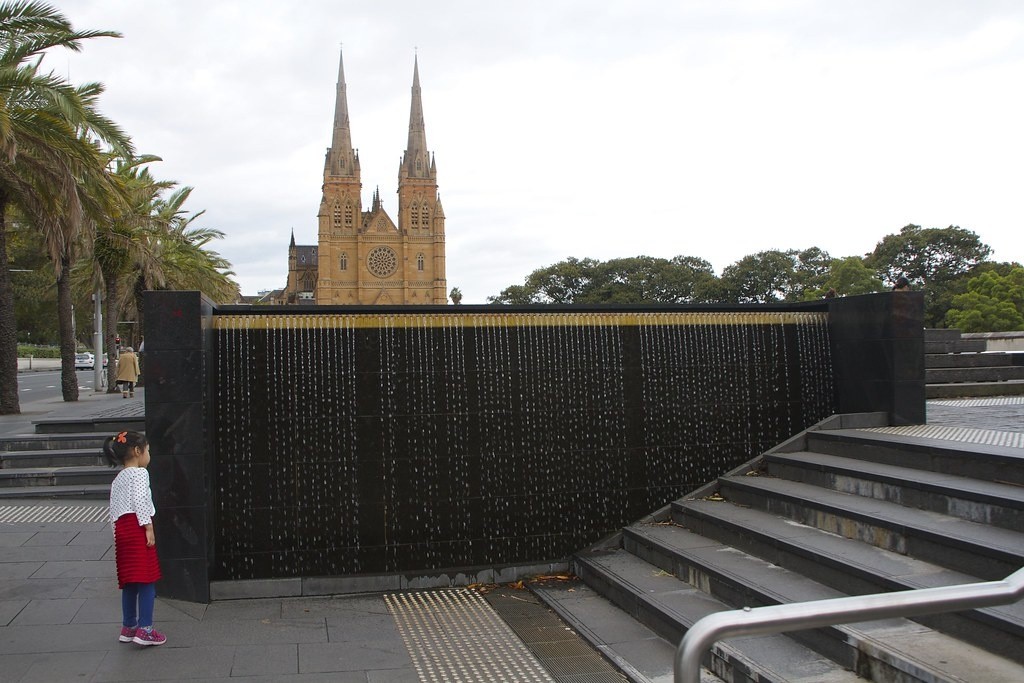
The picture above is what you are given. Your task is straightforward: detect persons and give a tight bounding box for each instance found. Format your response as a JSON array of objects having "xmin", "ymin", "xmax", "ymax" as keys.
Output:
[
  {"xmin": 115, "ymin": 347, "xmax": 138, "ymax": 398},
  {"xmin": 825, "ymin": 288, "xmax": 836, "ymax": 298},
  {"xmin": 104, "ymin": 431, "xmax": 166, "ymax": 645},
  {"xmin": 892, "ymin": 277, "xmax": 909, "ymax": 291}
]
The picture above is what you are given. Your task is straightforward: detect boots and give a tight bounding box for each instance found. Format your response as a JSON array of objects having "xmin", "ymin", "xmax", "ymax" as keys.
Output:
[
  {"xmin": 123, "ymin": 391, "xmax": 128, "ymax": 398},
  {"xmin": 130, "ymin": 392, "xmax": 134, "ymax": 399}
]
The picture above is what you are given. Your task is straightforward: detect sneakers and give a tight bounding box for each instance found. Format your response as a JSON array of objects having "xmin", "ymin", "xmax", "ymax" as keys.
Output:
[
  {"xmin": 119, "ymin": 627, "xmax": 138, "ymax": 642},
  {"xmin": 133, "ymin": 629, "xmax": 166, "ymax": 646}
]
